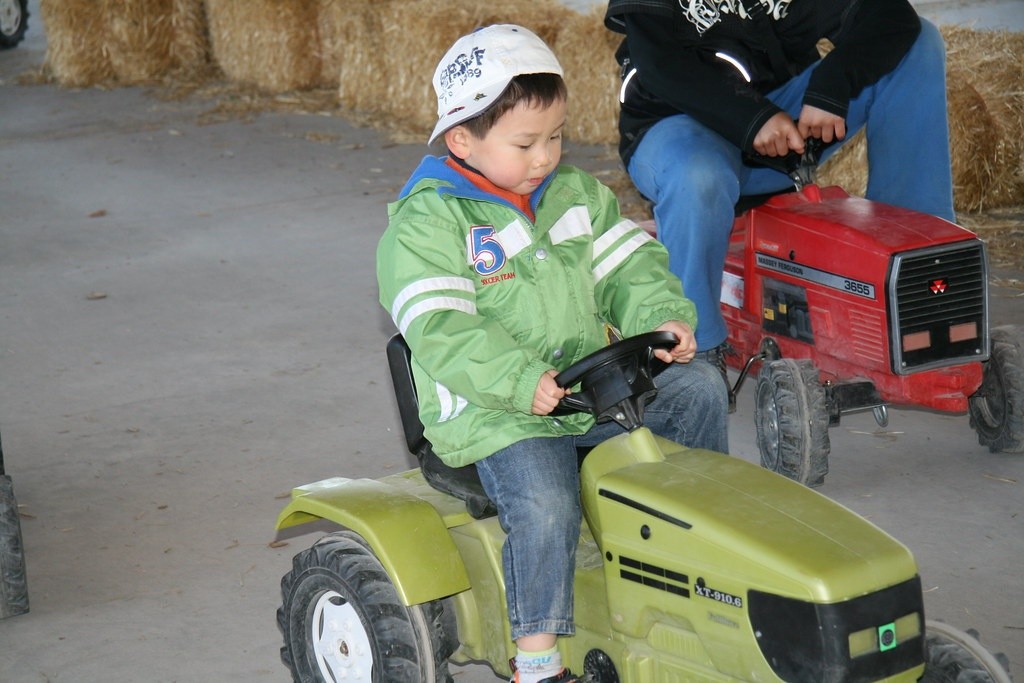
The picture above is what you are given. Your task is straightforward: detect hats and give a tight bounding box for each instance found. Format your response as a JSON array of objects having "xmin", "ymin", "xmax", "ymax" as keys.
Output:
[{"xmin": 428, "ymin": 24, "xmax": 563, "ymax": 147}]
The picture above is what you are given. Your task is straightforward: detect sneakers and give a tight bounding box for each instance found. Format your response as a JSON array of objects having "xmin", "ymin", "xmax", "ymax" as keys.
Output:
[
  {"xmin": 509, "ymin": 657, "xmax": 583, "ymax": 683},
  {"xmin": 695, "ymin": 341, "xmax": 737, "ymax": 414}
]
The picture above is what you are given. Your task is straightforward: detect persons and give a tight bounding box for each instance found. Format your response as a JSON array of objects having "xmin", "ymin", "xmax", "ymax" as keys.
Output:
[
  {"xmin": 604, "ymin": 0, "xmax": 957, "ymax": 413},
  {"xmin": 375, "ymin": 27, "xmax": 728, "ymax": 683}
]
